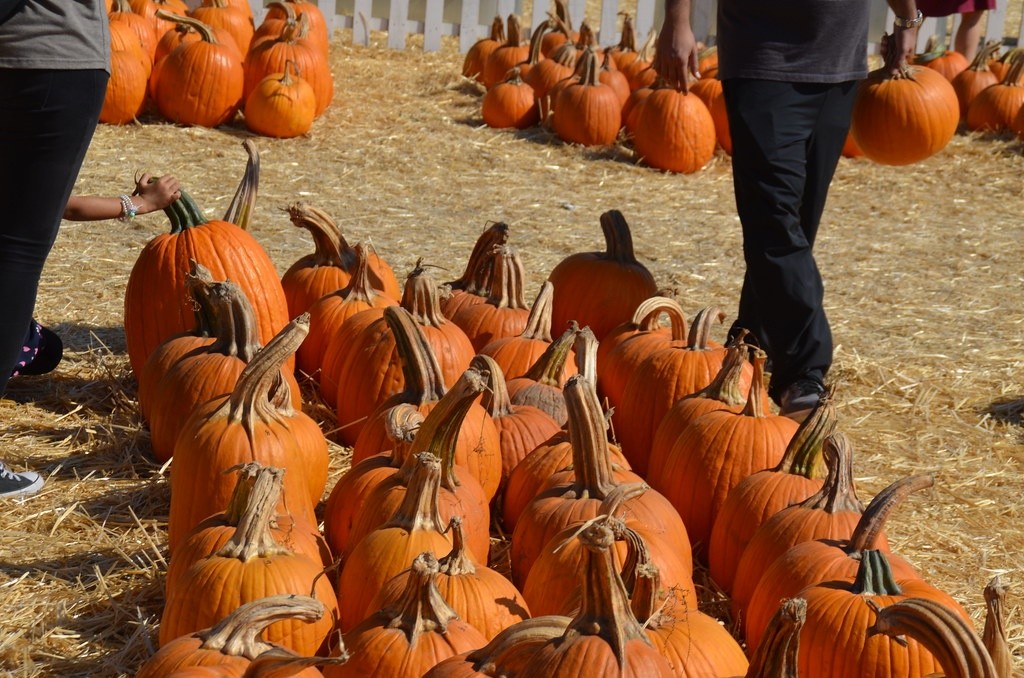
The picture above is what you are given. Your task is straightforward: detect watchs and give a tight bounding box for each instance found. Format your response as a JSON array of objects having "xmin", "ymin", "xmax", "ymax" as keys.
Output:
[{"xmin": 894, "ymin": 10, "xmax": 922, "ymax": 27}]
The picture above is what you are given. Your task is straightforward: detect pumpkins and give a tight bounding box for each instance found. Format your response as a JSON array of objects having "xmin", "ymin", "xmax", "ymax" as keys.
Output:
[
  {"xmin": 98, "ymin": 0, "xmax": 331, "ymax": 136},
  {"xmin": 121, "ymin": 136, "xmax": 1017, "ymax": 678},
  {"xmin": 462, "ymin": 0, "xmax": 1024, "ymax": 176}
]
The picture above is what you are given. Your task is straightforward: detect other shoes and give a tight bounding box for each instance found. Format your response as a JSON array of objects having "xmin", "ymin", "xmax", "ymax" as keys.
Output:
[{"xmin": 778, "ymin": 374, "xmax": 827, "ymax": 420}]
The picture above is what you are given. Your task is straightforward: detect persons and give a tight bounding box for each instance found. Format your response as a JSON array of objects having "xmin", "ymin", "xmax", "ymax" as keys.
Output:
[
  {"xmin": 0, "ymin": 0, "xmax": 181, "ymax": 496},
  {"xmin": 653, "ymin": 0, "xmax": 922, "ymax": 423},
  {"xmin": 916, "ymin": 0, "xmax": 997, "ymax": 65}
]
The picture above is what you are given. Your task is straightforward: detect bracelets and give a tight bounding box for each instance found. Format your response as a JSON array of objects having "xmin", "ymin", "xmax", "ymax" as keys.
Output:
[{"xmin": 118, "ymin": 196, "xmax": 138, "ymax": 223}]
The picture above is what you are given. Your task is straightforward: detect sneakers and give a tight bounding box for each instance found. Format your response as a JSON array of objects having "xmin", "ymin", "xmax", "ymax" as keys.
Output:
[{"xmin": 0, "ymin": 463, "xmax": 44, "ymax": 498}]
